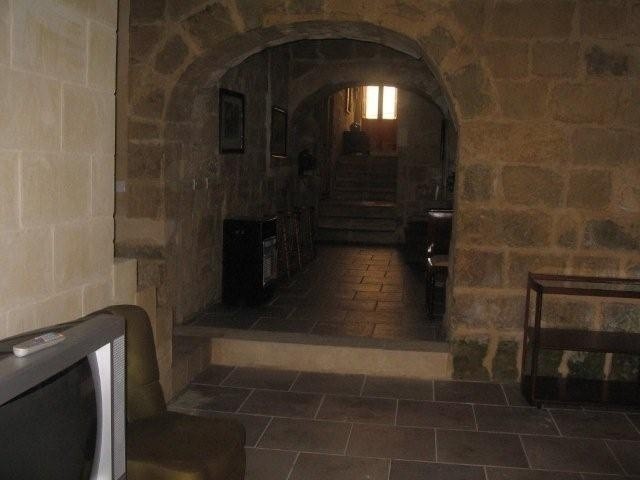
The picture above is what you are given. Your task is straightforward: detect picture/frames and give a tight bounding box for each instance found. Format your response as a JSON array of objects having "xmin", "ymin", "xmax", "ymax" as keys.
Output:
[
  {"xmin": 269, "ymin": 105, "xmax": 289, "ymax": 160},
  {"xmin": 218, "ymin": 87, "xmax": 246, "ymax": 156}
]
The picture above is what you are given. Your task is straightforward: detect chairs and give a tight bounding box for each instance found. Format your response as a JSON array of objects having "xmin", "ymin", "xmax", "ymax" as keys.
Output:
[
  {"xmin": 422, "ymin": 209, "xmax": 454, "ymax": 321},
  {"xmin": 81, "ymin": 303, "xmax": 248, "ymax": 480}
]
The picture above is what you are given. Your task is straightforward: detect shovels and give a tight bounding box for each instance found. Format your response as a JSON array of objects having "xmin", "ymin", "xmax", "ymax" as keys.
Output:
[{"xmin": 430, "ymin": 119, "xmax": 448, "ymax": 201}]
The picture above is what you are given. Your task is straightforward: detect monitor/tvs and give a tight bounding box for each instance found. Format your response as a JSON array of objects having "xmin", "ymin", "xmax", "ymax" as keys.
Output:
[{"xmin": 0, "ymin": 309, "xmax": 127, "ymax": 480}]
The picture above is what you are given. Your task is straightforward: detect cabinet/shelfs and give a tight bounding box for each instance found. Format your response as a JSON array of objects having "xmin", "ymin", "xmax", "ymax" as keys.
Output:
[
  {"xmin": 223, "ymin": 211, "xmax": 282, "ymax": 304},
  {"xmin": 521, "ymin": 271, "xmax": 639, "ymax": 409}
]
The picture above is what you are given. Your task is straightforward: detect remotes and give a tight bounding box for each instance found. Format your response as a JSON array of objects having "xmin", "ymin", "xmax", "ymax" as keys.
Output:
[{"xmin": 9, "ymin": 330, "xmax": 68, "ymax": 356}]
